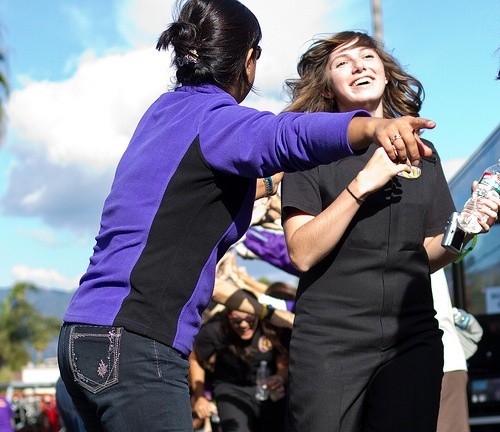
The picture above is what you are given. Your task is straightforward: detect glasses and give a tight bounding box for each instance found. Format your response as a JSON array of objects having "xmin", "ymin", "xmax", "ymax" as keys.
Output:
[
  {"xmin": 254, "ymin": 45, "xmax": 262, "ymax": 60},
  {"xmin": 228, "ymin": 316, "xmax": 256, "ymax": 325}
]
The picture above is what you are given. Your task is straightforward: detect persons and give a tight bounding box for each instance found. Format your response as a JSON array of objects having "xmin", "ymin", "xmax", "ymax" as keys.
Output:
[
  {"xmin": 57, "ymin": 0, "xmax": 437, "ymax": 432},
  {"xmin": 280, "ymin": 31, "xmax": 500, "ymax": 432},
  {"xmin": 0, "ymin": 192, "xmax": 483, "ymax": 432}
]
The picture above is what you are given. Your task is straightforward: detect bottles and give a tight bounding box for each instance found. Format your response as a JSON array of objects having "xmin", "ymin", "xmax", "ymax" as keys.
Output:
[
  {"xmin": 458, "ymin": 159, "xmax": 500, "ymax": 234},
  {"xmin": 255, "ymin": 361, "xmax": 270, "ymax": 400}
]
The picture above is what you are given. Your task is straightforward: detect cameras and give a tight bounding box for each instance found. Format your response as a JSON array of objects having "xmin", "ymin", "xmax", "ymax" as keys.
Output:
[{"xmin": 441, "ymin": 212, "xmax": 467, "ymax": 255}]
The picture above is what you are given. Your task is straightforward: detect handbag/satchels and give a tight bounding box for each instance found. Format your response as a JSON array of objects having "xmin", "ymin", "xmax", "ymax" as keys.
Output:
[{"xmin": 453, "ymin": 306, "xmax": 483, "ymax": 360}]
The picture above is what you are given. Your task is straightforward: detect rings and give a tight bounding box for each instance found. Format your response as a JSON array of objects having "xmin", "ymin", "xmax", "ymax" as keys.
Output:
[{"xmin": 390, "ymin": 134, "xmax": 402, "ymax": 144}]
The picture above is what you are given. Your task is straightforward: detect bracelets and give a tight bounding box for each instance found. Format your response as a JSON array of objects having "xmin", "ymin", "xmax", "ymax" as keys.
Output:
[
  {"xmin": 264, "ymin": 177, "xmax": 274, "ymax": 197},
  {"xmin": 346, "ymin": 186, "xmax": 365, "ymax": 202}
]
